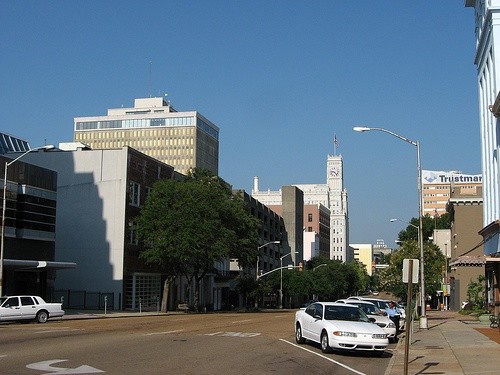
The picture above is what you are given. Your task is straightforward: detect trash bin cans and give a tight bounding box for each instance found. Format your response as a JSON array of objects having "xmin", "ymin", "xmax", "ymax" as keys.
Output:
[{"xmin": 417, "ymin": 306, "xmax": 426, "ymax": 316}]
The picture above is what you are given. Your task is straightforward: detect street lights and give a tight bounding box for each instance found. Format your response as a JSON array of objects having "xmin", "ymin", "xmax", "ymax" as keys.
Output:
[
  {"xmin": 312, "ymin": 263, "xmax": 327, "ymax": 300},
  {"xmin": 389, "ymin": 218, "xmax": 421, "ymax": 248},
  {"xmin": 350, "ymin": 125, "xmax": 430, "ymax": 330},
  {"xmin": 0, "ymin": 145, "xmax": 56, "ymax": 300},
  {"xmin": 255, "ymin": 240, "xmax": 280, "ymax": 308},
  {"xmin": 279, "ymin": 252, "xmax": 299, "ymax": 309}
]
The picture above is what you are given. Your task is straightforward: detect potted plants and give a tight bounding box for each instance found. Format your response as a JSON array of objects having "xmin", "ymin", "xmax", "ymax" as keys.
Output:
[{"xmin": 476, "ymin": 305, "xmax": 494, "ymax": 324}]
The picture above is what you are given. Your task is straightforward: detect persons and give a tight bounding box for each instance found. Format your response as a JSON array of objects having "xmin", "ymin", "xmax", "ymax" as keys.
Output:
[{"xmin": 376, "ymin": 301, "xmax": 400, "ymax": 335}]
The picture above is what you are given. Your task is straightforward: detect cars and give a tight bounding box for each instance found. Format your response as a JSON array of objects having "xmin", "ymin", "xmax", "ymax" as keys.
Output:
[
  {"xmin": 334, "ymin": 298, "xmax": 396, "ymax": 342},
  {"xmin": 0, "ymin": 295, "xmax": 65, "ymax": 324},
  {"xmin": 372, "ymin": 291, "xmax": 379, "ymax": 296},
  {"xmin": 348, "ymin": 295, "xmax": 407, "ymax": 335},
  {"xmin": 294, "ymin": 301, "xmax": 389, "ymax": 356}
]
